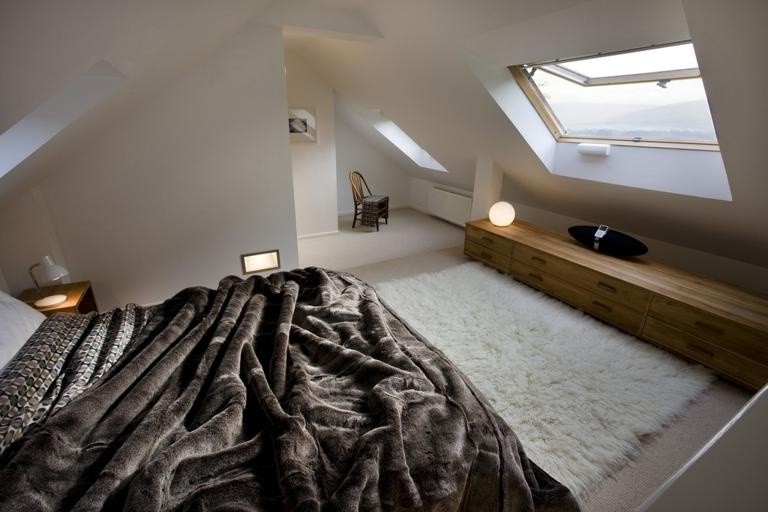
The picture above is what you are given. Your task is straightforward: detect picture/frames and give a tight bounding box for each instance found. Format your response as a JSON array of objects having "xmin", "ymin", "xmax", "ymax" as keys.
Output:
[{"xmin": 288, "ymin": 106, "xmax": 317, "ymax": 143}]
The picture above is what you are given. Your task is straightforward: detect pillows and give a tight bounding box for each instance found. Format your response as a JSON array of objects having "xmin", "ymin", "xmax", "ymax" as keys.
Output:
[{"xmin": 0, "ymin": 292, "xmax": 49, "ymax": 377}]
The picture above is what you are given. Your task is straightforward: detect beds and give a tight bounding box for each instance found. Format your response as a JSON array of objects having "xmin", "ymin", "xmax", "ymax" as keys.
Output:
[{"xmin": 0, "ymin": 267, "xmax": 579, "ymax": 512}]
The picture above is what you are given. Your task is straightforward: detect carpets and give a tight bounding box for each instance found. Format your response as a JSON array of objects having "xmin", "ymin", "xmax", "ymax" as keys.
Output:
[{"xmin": 370, "ymin": 259, "xmax": 718, "ymax": 504}]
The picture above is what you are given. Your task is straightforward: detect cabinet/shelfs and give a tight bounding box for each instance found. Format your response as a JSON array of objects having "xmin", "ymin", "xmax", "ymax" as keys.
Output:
[{"xmin": 461, "ymin": 217, "xmax": 767, "ymax": 395}]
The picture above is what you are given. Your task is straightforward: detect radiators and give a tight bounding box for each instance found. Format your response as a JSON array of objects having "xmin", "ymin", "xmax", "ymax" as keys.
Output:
[{"xmin": 428, "ymin": 186, "xmax": 472, "ymax": 229}]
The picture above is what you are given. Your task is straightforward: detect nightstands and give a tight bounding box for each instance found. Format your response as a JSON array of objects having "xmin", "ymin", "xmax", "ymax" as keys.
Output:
[{"xmin": 18, "ymin": 279, "xmax": 101, "ymax": 320}]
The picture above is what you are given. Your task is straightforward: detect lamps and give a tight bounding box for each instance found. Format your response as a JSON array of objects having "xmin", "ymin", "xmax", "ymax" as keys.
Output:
[
  {"xmin": 486, "ymin": 198, "xmax": 515, "ymax": 227},
  {"xmin": 25, "ymin": 254, "xmax": 72, "ymax": 309}
]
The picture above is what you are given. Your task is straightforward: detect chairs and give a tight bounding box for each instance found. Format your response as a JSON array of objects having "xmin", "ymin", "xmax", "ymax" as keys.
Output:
[{"xmin": 347, "ymin": 169, "xmax": 389, "ymax": 231}]
